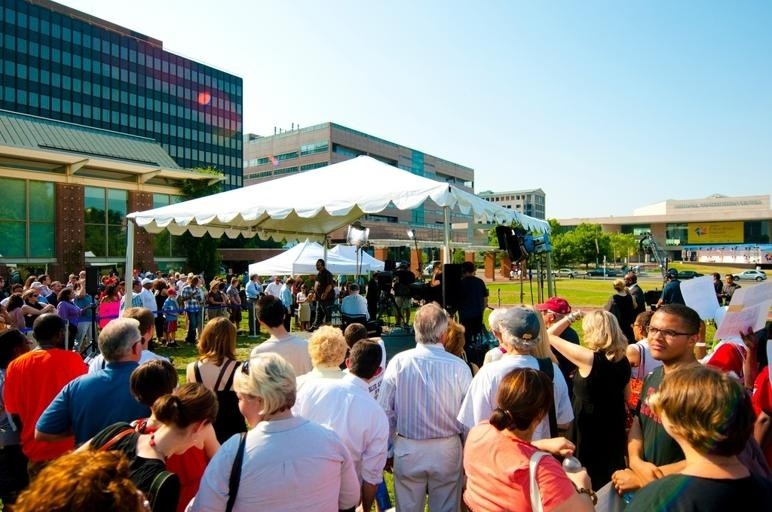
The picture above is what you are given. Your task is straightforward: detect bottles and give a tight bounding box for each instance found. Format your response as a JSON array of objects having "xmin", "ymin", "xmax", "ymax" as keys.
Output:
[
  {"xmin": 558, "ymin": 447, "xmax": 593, "ymax": 501},
  {"xmin": 622, "ymin": 490, "xmax": 635, "ymax": 504}
]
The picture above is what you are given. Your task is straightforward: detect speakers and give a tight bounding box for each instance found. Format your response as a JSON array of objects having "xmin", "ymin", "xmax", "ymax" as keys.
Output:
[
  {"xmin": 445, "ymin": 263, "xmax": 463, "ymax": 309},
  {"xmin": 85, "ymin": 266, "xmax": 100, "ymax": 295}
]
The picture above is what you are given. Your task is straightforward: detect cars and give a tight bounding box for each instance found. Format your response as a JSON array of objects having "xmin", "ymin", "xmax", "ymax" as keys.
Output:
[
  {"xmin": 542, "ymin": 265, "xmax": 644, "ymax": 278},
  {"xmin": 388, "ymin": 259, "xmax": 408, "ymax": 269},
  {"xmin": 732, "ymin": 269, "xmax": 768, "ymax": 283},
  {"xmin": 677, "ymin": 269, "xmax": 704, "ymax": 280},
  {"xmin": 425, "ymin": 259, "xmax": 441, "ymax": 274}
]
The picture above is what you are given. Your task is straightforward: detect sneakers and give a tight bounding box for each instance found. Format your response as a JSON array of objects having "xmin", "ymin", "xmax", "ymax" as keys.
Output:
[
  {"xmin": 306, "ymin": 325, "xmax": 318, "ymax": 332},
  {"xmin": 158, "ymin": 337, "xmax": 181, "ymax": 347}
]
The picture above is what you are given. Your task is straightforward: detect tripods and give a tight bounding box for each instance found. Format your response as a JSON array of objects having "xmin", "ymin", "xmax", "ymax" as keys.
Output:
[
  {"xmin": 79, "ymin": 295, "xmax": 99, "ymax": 355},
  {"xmin": 377, "ymin": 290, "xmax": 409, "ymax": 330}
]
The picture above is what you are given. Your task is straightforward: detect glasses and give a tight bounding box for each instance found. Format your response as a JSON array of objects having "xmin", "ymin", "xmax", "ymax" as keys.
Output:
[
  {"xmin": 241, "ymin": 361, "xmax": 249, "ymax": 376},
  {"xmin": 644, "ymin": 324, "xmax": 698, "ymax": 337},
  {"xmin": 131, "ymin": 336, "xmax": 146, "ymax": 349}
]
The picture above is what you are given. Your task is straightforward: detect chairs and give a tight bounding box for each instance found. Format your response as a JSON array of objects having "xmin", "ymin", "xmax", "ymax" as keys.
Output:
[{"xmin": 340, "ymin": 312, "xmax": 384, "ymax": 339}]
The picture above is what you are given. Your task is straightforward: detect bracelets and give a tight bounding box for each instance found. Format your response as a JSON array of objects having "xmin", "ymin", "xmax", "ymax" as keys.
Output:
[{"xmin": 577, "ymin": 488, "xmax": 598, "ymax": 506}]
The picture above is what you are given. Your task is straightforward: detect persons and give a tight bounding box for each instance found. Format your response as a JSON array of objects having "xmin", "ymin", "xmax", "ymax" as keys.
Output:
[
  {"xmin": 183, "ymin": 353, "xmax": 360, "ymax": 512},
  {"xmin": 2, "ymin": 259, "xmax": 771, "ymax": 493},
  {"xmin": 290, "ymin": 339, "xmax": 388, "ymax": 512},
  {"xmin": 624, "ymin": 364, "xmax": 771, "ymax": 512},
  {"xmin": 69, "ymin": 382, "xmax": 219, "ymax": 512},
  {"xmin": 462, "ymin": 367, "xmax": 598, "ymax": 512},
  {"xmin": 612, "ymin": 303, "xmax": 706, "ymax": 511},
  {"xmin": 5, "ymin": 447, "xmax": 153, "ymax": 512},
  {"xmin": 377, "ymin": 303, "xmax": 473, "ymax": 511}
]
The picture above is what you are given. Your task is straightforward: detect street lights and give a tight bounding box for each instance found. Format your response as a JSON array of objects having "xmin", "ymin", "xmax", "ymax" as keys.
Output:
[{"xmin": 407, "ymin": 227, "xmax": 422, "ymax": 273}]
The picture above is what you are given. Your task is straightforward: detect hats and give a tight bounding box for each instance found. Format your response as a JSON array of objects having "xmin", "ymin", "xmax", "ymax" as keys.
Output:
[
  {"xmin": 501, "ymin": 297, "xmax": 571, "ymax": 341},
  {"xmin": 350, "ymin": 283, "xmax": 360, "ymax": 291},
  {"xmin": 30, "ymin": 281, "xmax": 46, "ymax": 289},
  {"xmin": 141, "ymin": 271, "xmax": 154, "ymax": 285},
  {"xmin": 180, "ymin": 272, "xmax": 194, "ymax": 278},
  {"xmin": 69, "ymin": 274, "xmax": 78, "ymax": 278},
  {"xmin": 210, "ymin": 280, "xmax": 219, "ymax": 289}
]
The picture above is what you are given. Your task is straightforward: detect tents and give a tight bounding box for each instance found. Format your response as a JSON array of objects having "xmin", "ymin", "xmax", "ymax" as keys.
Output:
[{"xmin": 247, "ymin": 239, "xmax": 384, "ymax": 278}]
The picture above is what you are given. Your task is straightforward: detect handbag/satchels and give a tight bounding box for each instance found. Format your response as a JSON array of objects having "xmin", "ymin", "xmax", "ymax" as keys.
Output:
[{"xmin": 626, "ymin": 378, "xmax": 646, "ymax": 409}]
